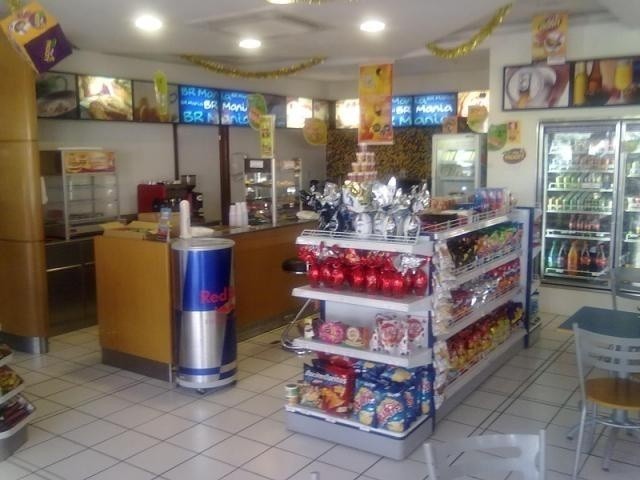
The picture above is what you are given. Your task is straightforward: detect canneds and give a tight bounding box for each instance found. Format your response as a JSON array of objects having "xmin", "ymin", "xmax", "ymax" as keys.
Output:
[
  {"xmin": 555, "ymin": 174, "xmax": 608, "ymax": 191},
  {"xmin": 549, "ymin": 194, "xmax": 612, "ymax": 212}
]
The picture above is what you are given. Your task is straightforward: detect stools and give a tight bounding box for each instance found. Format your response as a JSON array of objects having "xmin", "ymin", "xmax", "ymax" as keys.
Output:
[{"xmin": 282, "ymin": 258, "xmax": 316, "ymax": 355}]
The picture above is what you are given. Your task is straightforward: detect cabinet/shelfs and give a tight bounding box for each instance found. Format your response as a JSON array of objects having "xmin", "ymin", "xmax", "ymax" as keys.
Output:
[
  {"xmin": 243, "ymin": 155, "xmax": 302, "ymax": 218},
  {"xmin": 544, "ymin": 133, "xmax": 613, "ymax": 281},
  {"xmin": 283, "ymin": 207, "xmax": 543, "ymax": 460},
  {"xmin": 0, "ymin": 343, "xmax": 36, "ymax": 461},
  {"xmin": 622, "ymin": 131, "xmax": 640, "ymax": 270},
  {"xmin": 38, "ymin": 147, "xmax": 120, "ymax": 241}
]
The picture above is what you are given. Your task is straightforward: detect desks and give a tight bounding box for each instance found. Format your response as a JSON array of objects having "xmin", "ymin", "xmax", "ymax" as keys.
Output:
[{"xmin": 557, "ymin": 306, "xmax": 640, "ymax": 472}]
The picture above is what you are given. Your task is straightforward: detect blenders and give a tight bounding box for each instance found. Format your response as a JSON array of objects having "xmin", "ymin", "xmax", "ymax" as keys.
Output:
[{"xmin": 181, "ymin": 174, "xmax": 204, "ymax": 216}]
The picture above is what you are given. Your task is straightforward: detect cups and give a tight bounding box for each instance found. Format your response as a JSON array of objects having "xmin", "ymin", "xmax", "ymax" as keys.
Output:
[
  {"xmin": 229, "ymin": 202, "xmax": 248, "ymax": 227},
  {"xmin": 347, "ymin": 144, "xmax": 378, "ymax": 182}
]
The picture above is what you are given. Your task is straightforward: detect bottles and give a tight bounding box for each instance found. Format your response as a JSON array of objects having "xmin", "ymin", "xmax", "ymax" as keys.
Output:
[
  {"xmin": 474, "ymin": 186, "xmax": 508, "ymax": 214},
  {"xmin": 558, "ymin": 240, "xmax": 569, "ymax": 268},
  {"xmin": 567, "ymin": 214, "xmax": 576, "ymax": 234},
  {"xmin": 546, "ymin": 240, "xmax": 557, "ymax": 269},
  {"xmin": 577, "ymin": 215, "xmax": 584, "ymax": 236},
  {"xmin": 584, "ymin": 216, "xmax": 592, "ymax": 235},
  {"xmin": 593, "ymin": 215, "xmax": 600, "ymax": 233},
  {"xmin": 580, "ymin": 244, "xmax": 590, "ymax": 270},
  {"xmin": 569, "ymin": 240, "xmax": 578, "ymax": 275},
  {"xmin": 596, "ymin": 245, "xmax": 607, "ymax": 272}
]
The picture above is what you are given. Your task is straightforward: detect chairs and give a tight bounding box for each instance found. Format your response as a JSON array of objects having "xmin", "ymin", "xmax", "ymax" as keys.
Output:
[
  {"xmin": 423, "ymin": 429, "xmax": 546, "ymax": 480},
  {"xmin": 609, "ymin": 268, "xmax": 640, "ymax": 309},
  {"xmin": 571, "ymin": 322, "xmax": 640, "ymax": 480}
]
